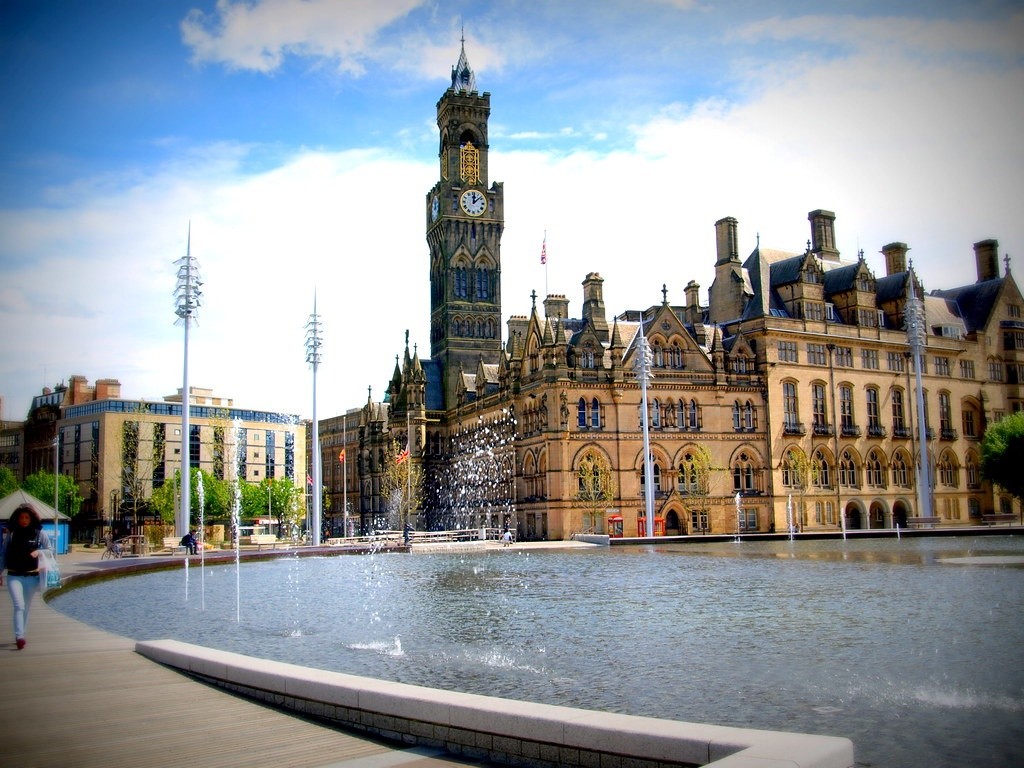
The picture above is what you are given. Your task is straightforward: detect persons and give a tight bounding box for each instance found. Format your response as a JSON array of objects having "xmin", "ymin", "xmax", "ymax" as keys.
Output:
[
  {"xmin": 182, "ymin": 529, "xmax": 199, "ymax": 555},
  {"xmin": 501, "ymin": 528, "xmax": 513, "ymax": 547},
  {"xmin": 0, "ymin": 503, "xmax": 53, "ymax": 651},
  {"xmin": 106, "ymin": 530, "xmax": 120, "ymax": 558}
]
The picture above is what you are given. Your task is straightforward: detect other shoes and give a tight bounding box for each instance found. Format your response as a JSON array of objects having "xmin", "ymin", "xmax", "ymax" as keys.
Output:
[{"xmin": 16, "ymin": 636, "xmax": 26, "ymax": 649}]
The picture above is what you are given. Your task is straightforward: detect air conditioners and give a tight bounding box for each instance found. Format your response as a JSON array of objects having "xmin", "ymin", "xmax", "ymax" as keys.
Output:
[{"xmin": 942, "ymin": 326, "xmax": 960, "ymax": 340}]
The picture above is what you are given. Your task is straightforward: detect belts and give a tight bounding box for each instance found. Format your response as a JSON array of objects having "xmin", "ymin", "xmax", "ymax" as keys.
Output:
[{"xmin": 7, "ymin": 571, "xmax": 39, "ymax": 576}]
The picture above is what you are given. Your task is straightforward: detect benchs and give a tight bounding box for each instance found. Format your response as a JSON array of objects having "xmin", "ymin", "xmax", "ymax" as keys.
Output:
[
  {"xmin": 981, "ymin": 514, "xmax": 1018, "ymax": 528},
  {"xmin": 163, "ymin": 536, "xmax": 195, "ymax": 557},
  {"xmin": 906, "ymin": 517, "xmax": 942, "ymax": 529},
  {"xmin": 250, "ymin": 534, "xmax": 283, "ymax": 550}
]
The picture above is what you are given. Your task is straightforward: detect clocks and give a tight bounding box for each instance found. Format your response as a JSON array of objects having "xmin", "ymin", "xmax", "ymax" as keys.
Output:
[{"xmin": 461, "ymin": 189, "xmax": 488, "ymax": 217}]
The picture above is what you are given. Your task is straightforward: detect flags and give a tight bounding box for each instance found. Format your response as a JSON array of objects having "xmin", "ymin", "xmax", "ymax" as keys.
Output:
[
  {"xmin": 541, "ymin": 239, "xmax": 547, "ymax": 265},
  {"xmin": 396, "ymin": 444, "xmax": 408, "ymax": 466},
  {"xmin": 307, "ymin": 473, "xmax": 312, "ymax": 486},
  {"xmin": 338, "ymin": 449, "xmax": 344, "ymax": 464}
]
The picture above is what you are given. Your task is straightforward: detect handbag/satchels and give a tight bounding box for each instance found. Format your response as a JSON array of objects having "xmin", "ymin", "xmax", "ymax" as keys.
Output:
[{"xmin": 38, "ymin": 549, "xmax": 62, "ymax": 593}]
